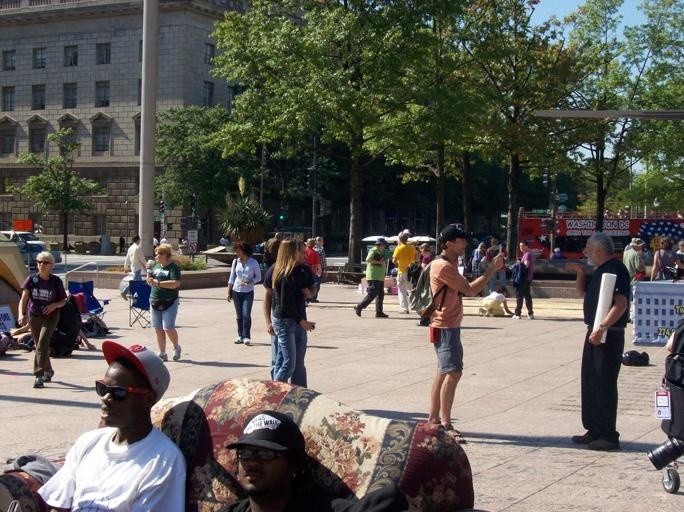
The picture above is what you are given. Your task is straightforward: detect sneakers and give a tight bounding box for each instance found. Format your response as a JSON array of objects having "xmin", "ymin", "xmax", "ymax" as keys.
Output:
[
  {"xmin": 157, "ymin": 352, "xmax": 167, "ymax": 360},
  {"xmin": 572, "ymin": 434, "xmax": 593, "ymax": 444},
  {"xmin": 241, "ymin": 340, "xmax": 250, "ymax": 346},
  {"xmin": 235, "ymin": 337, "xmax": 243, "ymax": 344},
  {"xmin": 33, "ymin": 376, "xmax": 43, "ymax": 387},
  {"xmin": 588, "ymin": 438, "xmax": 620, "ymax": 450},
  {"xmin": 173, "ymin": 346, "xmax": 181, "ymax": 360},
  {"xmin": 42, "ymin": 368, "xmax": 54, "ymax": 382}
]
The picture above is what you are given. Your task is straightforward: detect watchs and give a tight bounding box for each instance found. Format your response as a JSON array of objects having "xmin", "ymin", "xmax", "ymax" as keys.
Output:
[{"xmin": 155, "ymin": 281, "xmax": 160, "ymax": 288}]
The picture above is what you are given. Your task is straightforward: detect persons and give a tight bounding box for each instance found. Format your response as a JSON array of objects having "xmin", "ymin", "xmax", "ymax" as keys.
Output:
[
  {"xmin": 153, "ymin": 236, "xmax": 167, "ymax": 248},
  {"xmin": 145, "ymin": 243, "xmax": 182, "ymax": 361},
  {"xmin": 120, "ymin": 237, "xmax": 127, "ymax": 253},
  {"xmin": 0, "ymin": 338, "xmax": 188, "ymax": 511},
  {"xmin": 354, "ymin": 232, "xmax": 433, "ymax": 327},
  {"xmin": 216, "ymin": 409, "xmax": 409, "ymax": 511},
  {"xmin": 226, "ymin": 240, "xmax": 262, "ymax": 345},
  {"xmin": 658, "ymin": 322, "xmax": 684, "ymax": 442},
  {"xmin": 621, "ymin": 235, "xmax": 684, "ymax": 323},
  {"xmin": 426, "ymin": 223, "xmax": 505, "ymax": 446},
  {"xmin": 119, "ymin": 235, "xmax": 150, "ymax": 301},
  {"xmin": 17, "ymin": 250, "xmax": 68, "ymax": 388},
  {"xmin": 263, "ymin": 231, "xmax": 326, "ymax": 388},
  {"xmin": 469, "ymin": 236, "xmax": 536, "ymax": 320},
  {"xmin": 564, "ymin": 231, "xmax": 631, "ymax": 449},
  {"xmin": 550, "ymin": 248, "xmax": 567, "ymax": 259}
]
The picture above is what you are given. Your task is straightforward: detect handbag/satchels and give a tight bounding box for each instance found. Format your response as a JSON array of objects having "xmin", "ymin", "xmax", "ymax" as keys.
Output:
[
  {"xmin": 230, "ymin": 285, "xmax": 233, "ymax": 298},
  {"xmin": 151, "ymin": 297, "xmax": 174, "ymax": 311},
  {"xmin": 662, "ymin": 265, "xmax": 678, "ymax": 280}
]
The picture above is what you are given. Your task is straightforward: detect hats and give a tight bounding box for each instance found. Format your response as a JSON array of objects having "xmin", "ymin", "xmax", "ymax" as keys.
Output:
[
  {"xmin": 226, "ymin": 411, "xmax": 305, "ymax": 451},
  {"xmin": 630, "ymin": 237, "xmax": 645, "ymax": 247},
  {"xmin": 102, "ymin": 341, "xmax": 170, "ymax": 402},
  {"xmin": 375, "ymin": 238, "xmax": 387, "ymax": 244},
  {"xmin": 439, "ymin": 223, "xmax": 468, "ymax": 239}
]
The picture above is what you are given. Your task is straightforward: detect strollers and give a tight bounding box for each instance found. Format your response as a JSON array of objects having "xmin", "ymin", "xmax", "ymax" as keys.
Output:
[{"xmin": 648, "ymin": 321, "xmax": 684, "ymax": 494}]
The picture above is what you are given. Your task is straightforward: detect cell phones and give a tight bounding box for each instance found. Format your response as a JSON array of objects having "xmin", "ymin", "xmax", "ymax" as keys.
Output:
[{"xmin": 498, "ymin": 244, "xmax": 504, "ymax": 254}]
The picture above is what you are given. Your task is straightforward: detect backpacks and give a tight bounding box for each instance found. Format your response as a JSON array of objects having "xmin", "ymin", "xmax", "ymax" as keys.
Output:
[{"xmin": 406, "ymin": 255, "xmax": 452, "ymax": 318}]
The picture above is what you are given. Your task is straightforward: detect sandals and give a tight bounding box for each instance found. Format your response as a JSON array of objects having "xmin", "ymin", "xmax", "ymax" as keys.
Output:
[{"xmin": 445, "ymin": 425, "xmax": 465, "ymax": 444}]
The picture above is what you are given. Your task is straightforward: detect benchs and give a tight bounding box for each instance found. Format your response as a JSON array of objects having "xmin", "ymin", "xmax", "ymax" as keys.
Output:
[
  {"xmin": 0, "ymin": 377, "xmax": 474, "ymax": 512},
  {"xmin": 337, "ymin": 262, "xmax": 366, "ymax": 285}
]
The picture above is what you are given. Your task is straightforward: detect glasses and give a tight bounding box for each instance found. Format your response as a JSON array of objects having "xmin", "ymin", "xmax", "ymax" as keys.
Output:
[
  {"xmin": 236, "ymin": 448, "xmax": 283, "ymax": 460},
  {"xmin": 95, "ymin": 379, "xmax": 149, "ymax": 401},
  {"xmin": 37, "ymin": 261, "xmax": 50, "ymax": 264}
]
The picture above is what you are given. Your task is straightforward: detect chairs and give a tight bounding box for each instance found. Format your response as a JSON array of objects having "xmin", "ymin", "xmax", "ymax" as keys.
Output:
[
  {"xmin": 129, "ymin": 280, "xmax": 151, "ymax": 328},
  {"xmin": 68, "ymin": 280, "xmax": 106, "ymax": 322}
]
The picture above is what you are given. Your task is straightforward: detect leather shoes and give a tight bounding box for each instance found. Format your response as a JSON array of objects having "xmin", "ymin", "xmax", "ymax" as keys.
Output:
[{"xmin": 354, "ymin": 305, "xmax": 361, "ymax": 316}]
